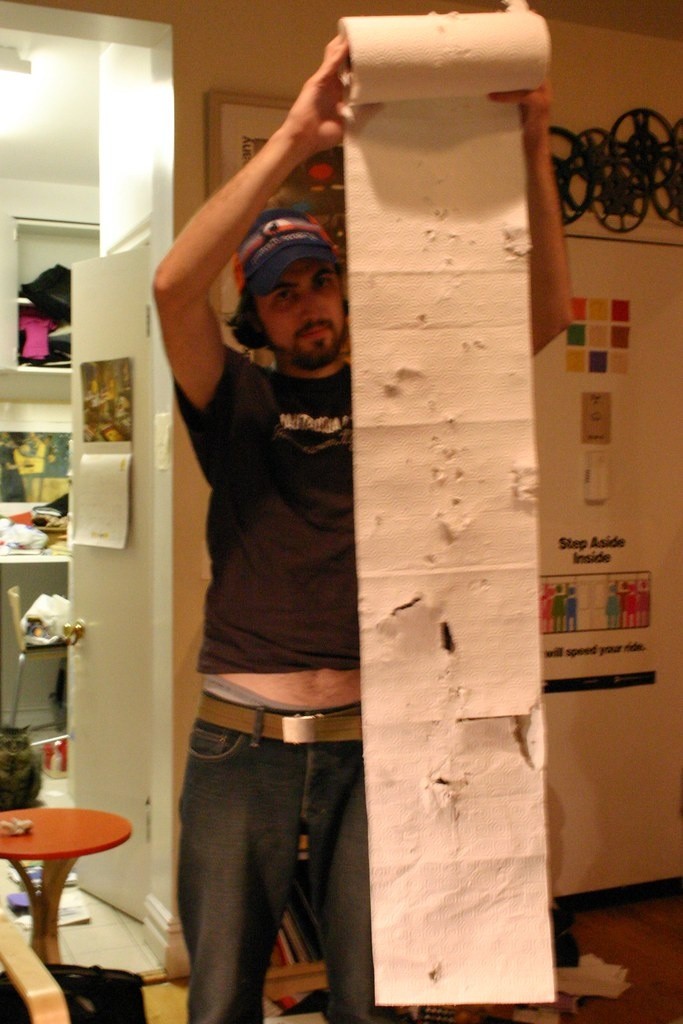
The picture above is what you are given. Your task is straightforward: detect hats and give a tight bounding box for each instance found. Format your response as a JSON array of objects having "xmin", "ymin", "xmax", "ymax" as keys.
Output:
[{"xmin": 233, "ymin": 208, "xmax": 338, "ymax": 296}]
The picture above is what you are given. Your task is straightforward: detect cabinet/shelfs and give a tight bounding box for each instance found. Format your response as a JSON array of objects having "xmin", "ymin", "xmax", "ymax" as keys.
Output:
[{"xmin": 14, "ymin": 217, "xmax": 101, "ymax": 397}]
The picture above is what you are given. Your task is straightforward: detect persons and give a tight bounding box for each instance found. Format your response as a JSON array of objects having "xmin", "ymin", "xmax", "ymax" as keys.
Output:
[{"xmin": 152, "ymin": 28, "xmax": 577, "ymax": 1023}]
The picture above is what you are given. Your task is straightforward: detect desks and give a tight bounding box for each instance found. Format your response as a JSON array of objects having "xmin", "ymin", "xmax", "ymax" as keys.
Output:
[
  {"xmin": 0, "ymin": 807, "xmax": 131, "ymax": 964},
  {"xmin": 0, "ymin": 557, "xmax": 68, "ymax": 564}
]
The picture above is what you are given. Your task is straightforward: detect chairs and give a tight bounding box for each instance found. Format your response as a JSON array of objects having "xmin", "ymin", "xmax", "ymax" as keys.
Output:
[{"xmin": 6, "ymin": 586, "xmax": 67, "ymax": 724}]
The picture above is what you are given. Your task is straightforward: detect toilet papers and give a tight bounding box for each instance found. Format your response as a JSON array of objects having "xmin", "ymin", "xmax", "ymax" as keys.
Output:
[{"xmin": 336, "ymin": 6, "xmax": 551, "ymax": 111}]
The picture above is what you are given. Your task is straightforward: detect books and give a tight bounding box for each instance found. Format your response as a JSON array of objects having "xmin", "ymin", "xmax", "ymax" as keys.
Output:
[{"xmin": 267, "ymin": 913, "xmax": 316, "ymax": 965}]
